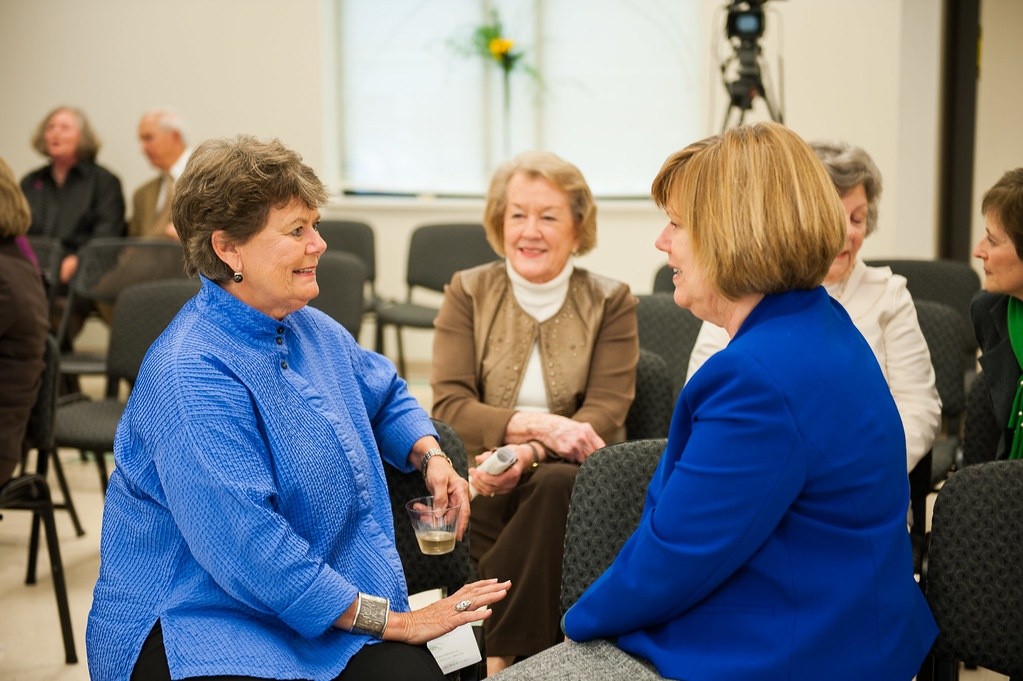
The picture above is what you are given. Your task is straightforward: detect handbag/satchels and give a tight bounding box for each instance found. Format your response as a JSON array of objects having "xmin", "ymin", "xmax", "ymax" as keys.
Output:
[{"xmin": 0, "ymin": 475, "xmax": 51, "ymax": 511}]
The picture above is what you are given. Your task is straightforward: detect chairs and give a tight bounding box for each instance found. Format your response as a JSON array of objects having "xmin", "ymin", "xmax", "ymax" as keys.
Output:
[{"xmin": 0, "ymin": 221, "xmax": 1022, "ymax": 681}]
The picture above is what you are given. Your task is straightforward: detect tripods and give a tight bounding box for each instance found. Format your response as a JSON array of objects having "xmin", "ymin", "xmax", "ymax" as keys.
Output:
[{"xmin": 722, "ymin": 37, "xmax": 778, "ymax": 138}]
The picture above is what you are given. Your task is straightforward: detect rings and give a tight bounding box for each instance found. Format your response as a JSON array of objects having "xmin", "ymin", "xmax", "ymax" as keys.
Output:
[{"xmin": 454, "ymin": 600, "xmax": 472, "ymax": 612}]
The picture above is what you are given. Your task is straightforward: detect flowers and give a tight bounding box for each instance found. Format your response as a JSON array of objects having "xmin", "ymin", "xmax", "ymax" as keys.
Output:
[{"xmin": 447, "ymin": 5, "xmax": 542, "ymax": 135}]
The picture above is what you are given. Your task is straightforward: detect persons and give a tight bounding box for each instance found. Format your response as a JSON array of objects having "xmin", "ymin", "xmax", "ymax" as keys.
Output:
[
  {"xmin": 13, "ymin": 104, "xmax": 124, "ymax": 402},
  {"xmin": 486, "ymin": 125, "xmax": 939, "ymax": 678},
  {"xmin": 682, "ymin": 135, "xmax": 944, "ymax": 532},
  {"xmin": 425, "ymin": 149, "xmax": 642, "ymax": 675},
  {"xmin": 86, "ymin": 132, "xmax": 513, "ymax": 681},
  {"xmin": 86, "ymin": 110, "xmax": 198, "ymax": 338},
  {"xmin": 970, "ymin": 169, "xmax": 1023, "ymax": 461},
  {"xmin": 0, "ymin": 157, "xmax": 54, "ymax": 487}
]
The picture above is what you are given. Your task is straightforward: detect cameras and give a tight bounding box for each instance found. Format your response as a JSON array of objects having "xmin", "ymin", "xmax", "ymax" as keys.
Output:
[{"xmin": 727, "ymin": 9, "xmax": 765, "ymax": 39}]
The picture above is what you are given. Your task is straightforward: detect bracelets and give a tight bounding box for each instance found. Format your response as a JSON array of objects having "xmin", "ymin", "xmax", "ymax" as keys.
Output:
[
  {"xmin": 420, "ymin": 448, "xmax": 454, "ymax": 478},
  {"xmin": 527, "ymin": 441, "xmax": 539, "ymax": 467},
  {"xmin": 348, "ymin": 592, "xmax": 391, "ymax": 640}
]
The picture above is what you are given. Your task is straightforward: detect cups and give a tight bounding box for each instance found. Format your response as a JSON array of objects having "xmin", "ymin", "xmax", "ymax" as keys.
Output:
[{"xmin": 405, "ymin": 496, "xmax": 461, "ymax": 556}]
